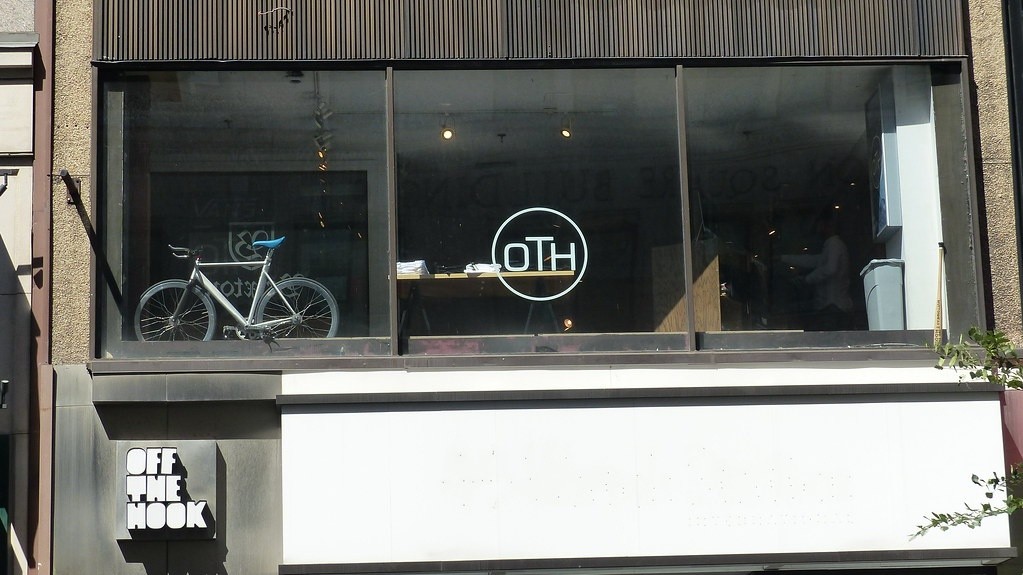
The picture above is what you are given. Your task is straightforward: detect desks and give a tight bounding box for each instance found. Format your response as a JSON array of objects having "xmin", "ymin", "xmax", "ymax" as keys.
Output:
[{"xmin": 388, "ymin": 268, "xmax": 574, "ymax": 356}]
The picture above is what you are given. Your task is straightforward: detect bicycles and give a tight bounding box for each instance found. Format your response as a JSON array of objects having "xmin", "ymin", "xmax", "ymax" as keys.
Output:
[{"xmin": 136, "ymin": 236, "xmax": 338, "ymax": 343}]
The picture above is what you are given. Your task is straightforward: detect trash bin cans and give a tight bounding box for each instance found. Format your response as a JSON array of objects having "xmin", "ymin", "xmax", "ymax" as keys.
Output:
[{"xmin": 860, "ymin": 258, "xmax": 904, "ymax": 331}]
